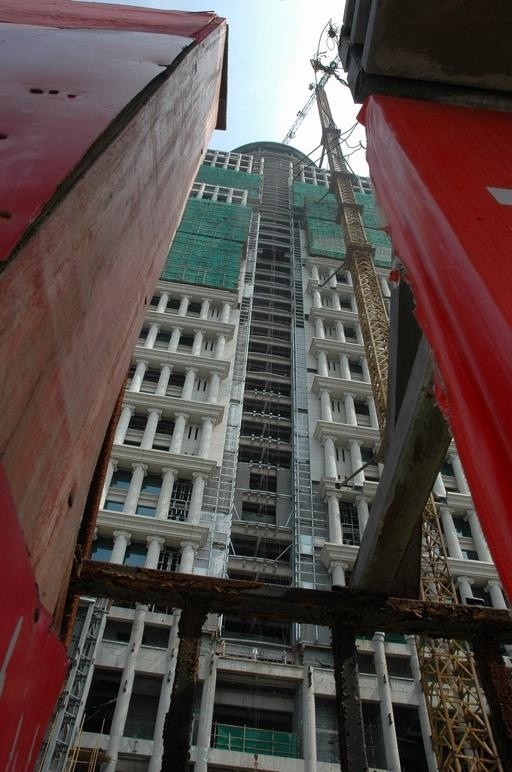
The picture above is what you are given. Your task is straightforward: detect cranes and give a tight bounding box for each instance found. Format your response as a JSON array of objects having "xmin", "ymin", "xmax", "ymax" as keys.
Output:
[{"xmin": 283, "ymin": 20, "xmax": 504, "ymax": 772}]
